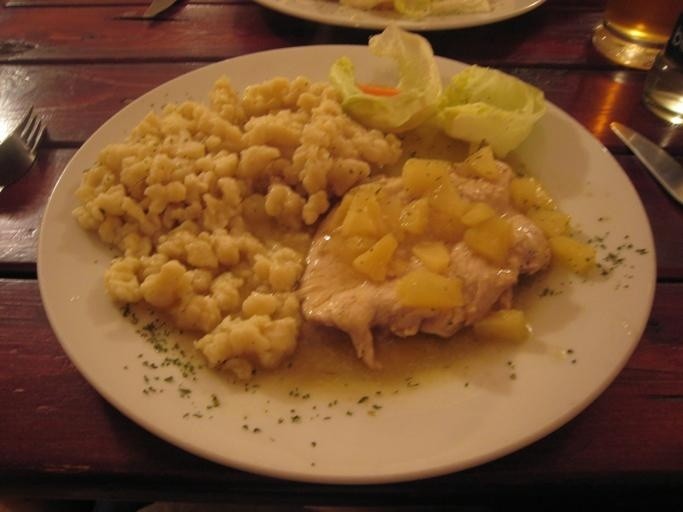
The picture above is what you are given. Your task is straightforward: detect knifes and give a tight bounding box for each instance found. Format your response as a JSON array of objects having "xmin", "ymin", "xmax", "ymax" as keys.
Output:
[
  {"xmin": 609, "ymin": 119, "xmax": 683, "ymax": 205},
  {"xmin": 139, "ymin": 0, "xmax": 178, "ymax": 19}
]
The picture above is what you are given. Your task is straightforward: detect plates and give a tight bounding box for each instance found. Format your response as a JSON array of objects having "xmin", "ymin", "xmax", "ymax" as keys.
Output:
[
  {"xmin": 255, "ymin": 1, "xmax": 548, "ymax": 29},
  {"xmin": 37, "ymin": 46, "xmax": 658, "ymax": 486}
]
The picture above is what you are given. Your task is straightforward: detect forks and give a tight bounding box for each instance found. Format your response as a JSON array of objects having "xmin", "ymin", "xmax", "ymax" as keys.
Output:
[{"xmin": 0, "ymin": 105, "xmax": 48, "ymax": 193}]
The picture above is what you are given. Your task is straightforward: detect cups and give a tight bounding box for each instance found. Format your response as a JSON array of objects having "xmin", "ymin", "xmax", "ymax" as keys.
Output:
[
  {"xmin": 640, "ymin": 14, "xmax": 683, "ymax": 126},
  {"xmin": 591, "ymin": 0, "xmax": 683, "ymax": 71}
]
